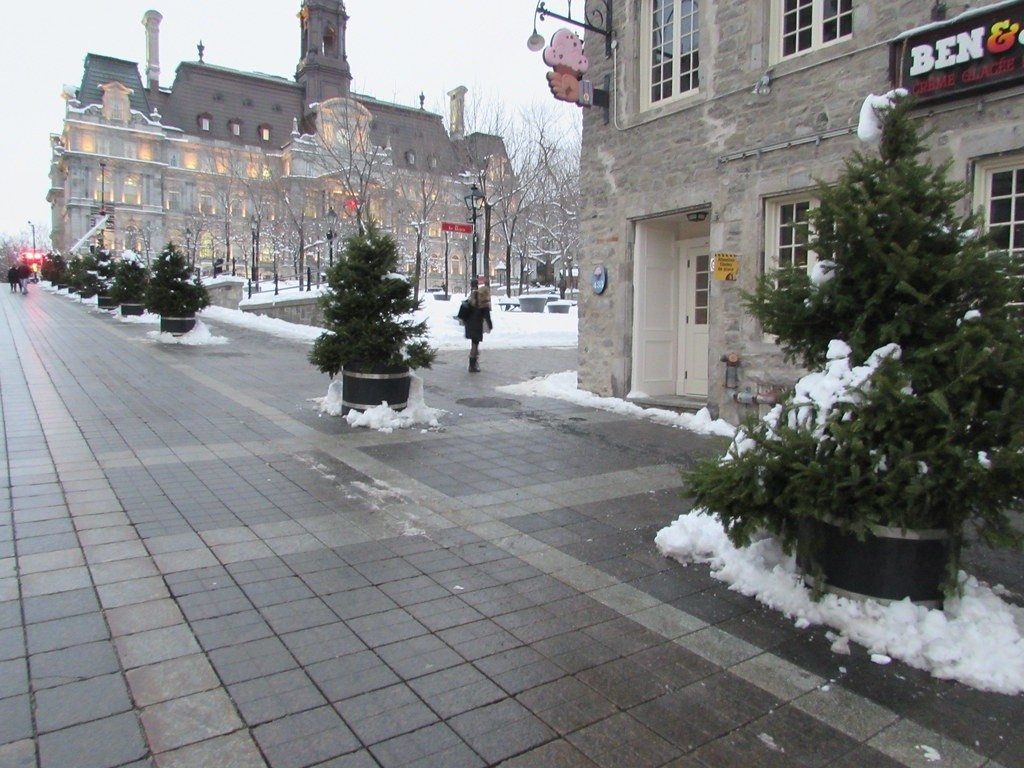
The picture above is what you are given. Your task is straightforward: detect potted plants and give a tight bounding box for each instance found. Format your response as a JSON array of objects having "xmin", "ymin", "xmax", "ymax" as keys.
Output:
[
  {"xmin": 306, "ymin": 212, "xmax": 440, "ymax": 417},
  {"xmin": 667, "ymin": 90, "xmax": 1024, "ymax": 609},
  {"xmin": 40, "ymin": 241, "xmax": 214, "ymax": 337}
]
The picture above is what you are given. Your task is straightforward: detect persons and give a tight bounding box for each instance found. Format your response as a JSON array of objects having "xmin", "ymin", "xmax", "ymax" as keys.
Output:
[
  {"xmin": 558, "ymin": 276, "xmax": 566, "ymax": 298},
  {"xmin": 458, "ymin": 286, "xmax": 492, "ymax": 372},
  {"xmin": 8, "ymin": 262, "xmax": 30, "ymax": 294},
  {"xmin": 214, "ymin": 259, "xmax": 223, "ymax": 274}
]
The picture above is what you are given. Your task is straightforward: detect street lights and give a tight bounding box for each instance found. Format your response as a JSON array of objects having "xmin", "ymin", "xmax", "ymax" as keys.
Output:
[{"xmin": 464, "ymin": 183, "xmax": 485, "ymax": 276}]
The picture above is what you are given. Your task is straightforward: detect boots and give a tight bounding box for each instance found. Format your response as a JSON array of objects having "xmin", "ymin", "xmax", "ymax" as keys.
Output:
[{"xmin": 469, "ymin": 357, "xmax": 480, "ymax": 372}]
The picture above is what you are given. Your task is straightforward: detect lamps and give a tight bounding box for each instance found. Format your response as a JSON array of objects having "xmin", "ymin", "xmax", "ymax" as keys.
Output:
[
  {"xmin": 527, "ymin": 0, "xmax": 614, "ymax": 57},
  {"xmin": 687, "ymin": 212, "xmax": 706, "ymax": 222},
  {"xmin": 743, "ymin": 67, "xmax": 773, "ymax": 105}
]
